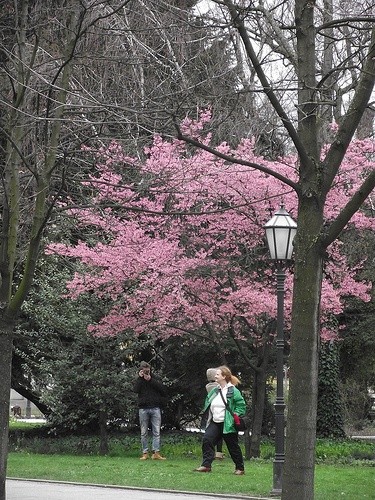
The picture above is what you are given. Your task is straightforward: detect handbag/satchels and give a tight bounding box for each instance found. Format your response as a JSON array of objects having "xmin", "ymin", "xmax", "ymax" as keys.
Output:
[{"xmin": 234, "ymin": 414, "xmax": 247, "ymax": 431}]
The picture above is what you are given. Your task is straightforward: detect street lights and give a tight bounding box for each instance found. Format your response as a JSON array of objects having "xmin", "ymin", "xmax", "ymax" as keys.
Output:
[{"xmin": 263, "ymin": 200, "xmax": 297, "ymax": 497}]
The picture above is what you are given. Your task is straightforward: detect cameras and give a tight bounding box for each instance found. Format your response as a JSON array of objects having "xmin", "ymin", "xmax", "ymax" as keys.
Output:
[{"xmin": 143, "ymin": 369, "xmax": 150, "ymax": 375}]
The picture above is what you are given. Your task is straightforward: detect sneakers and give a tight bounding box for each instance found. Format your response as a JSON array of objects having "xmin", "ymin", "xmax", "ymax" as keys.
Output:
[
  {"xmin": 140, "ymin": 453, "xmax": 149, "ymax": 460},
  {"xmin": 152, "ymin": 451, "xmax": 166, "ymax": 460}
]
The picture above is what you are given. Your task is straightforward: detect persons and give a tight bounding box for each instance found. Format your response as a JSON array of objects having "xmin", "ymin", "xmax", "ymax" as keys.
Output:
[
  {"xmin": 195, "ymin": 365, "xmax": 247, "ymax": 475},
  {"xmin": 133, "ymin": 362, "xmax": 167, "ymax": 460}
]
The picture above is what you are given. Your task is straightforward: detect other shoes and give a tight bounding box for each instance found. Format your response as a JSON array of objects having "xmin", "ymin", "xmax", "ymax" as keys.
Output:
[
  {"xmin": 194, "ymin": 466, "xmax": 211, "ymax": 472},
  {"xmin": 234, "ymin": 470, "xmax": 244, "ymax": 475},
  {"xmin": 216, "ymin": 452, "xmax": 224, "ymax": 461}
]
